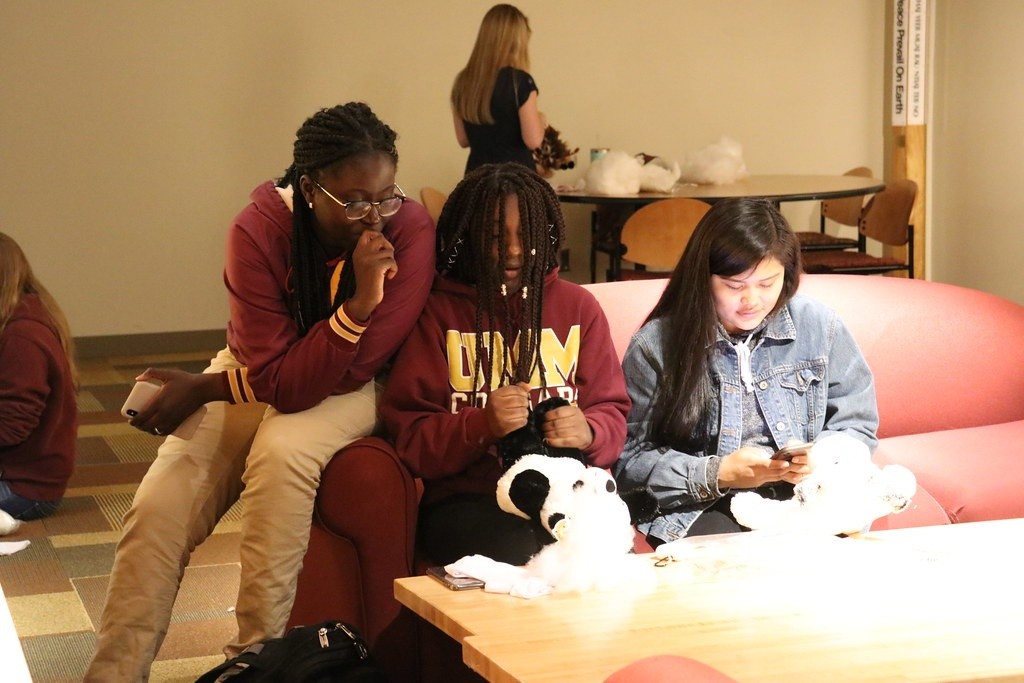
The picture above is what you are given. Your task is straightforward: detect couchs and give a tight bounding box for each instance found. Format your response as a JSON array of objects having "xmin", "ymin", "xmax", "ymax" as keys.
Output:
[{"xmin": 283, "ymin": 274, "xmax": 1024, "ymax": 682}]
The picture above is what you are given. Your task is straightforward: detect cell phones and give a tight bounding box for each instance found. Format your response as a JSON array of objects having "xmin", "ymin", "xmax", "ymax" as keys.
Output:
[
  {"xmin": 427, "ymin": 568, "xmax": 486, "ymax": 592},
  {"xmin": 769, "ymin": 443, "xmax": 815, "ymax": 461},
  {"xmin": 121, "ymin": 380, "xmax": 209, "ymax": 440}
]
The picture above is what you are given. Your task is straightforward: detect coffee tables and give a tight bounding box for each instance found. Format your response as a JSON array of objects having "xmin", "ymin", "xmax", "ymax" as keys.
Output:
[{"xmin": 390, "ymin": 519, "xmax": 1024, "ymax": 682}]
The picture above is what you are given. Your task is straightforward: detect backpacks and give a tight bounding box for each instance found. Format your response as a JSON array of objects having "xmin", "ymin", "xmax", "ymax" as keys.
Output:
[{"xmin": 191, "ymin": 620, "xmax": 382, "ymax": 683}]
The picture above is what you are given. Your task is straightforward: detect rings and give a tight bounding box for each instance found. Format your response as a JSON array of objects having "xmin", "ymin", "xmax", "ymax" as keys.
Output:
[{"xmin": 155, "ymin": 428, "xmax": 165, "ymax": 435}]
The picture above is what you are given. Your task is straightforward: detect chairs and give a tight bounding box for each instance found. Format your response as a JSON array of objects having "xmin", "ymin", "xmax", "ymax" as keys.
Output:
[
  {"xmin": 801, "ymin": 178, "xmax": 918, "ymax": 279},
  {"xmin": 794, "ymin": 167, "xmax": 874, "ymax": 251},
  {"xmin": 610, "ymin": 198, "xmax": 712, "ymax": 281}
]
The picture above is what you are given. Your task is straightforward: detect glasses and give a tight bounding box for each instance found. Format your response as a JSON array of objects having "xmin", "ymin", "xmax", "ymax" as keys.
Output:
[{"xmin": 312, "ymin": 180, "xmax": 408, "ymax": 221}]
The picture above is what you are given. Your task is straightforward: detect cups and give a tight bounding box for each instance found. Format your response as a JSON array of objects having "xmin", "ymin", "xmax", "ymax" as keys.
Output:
[{"xmin": 590, "ymin": 147, "xmax": 610, "ymax": 163}]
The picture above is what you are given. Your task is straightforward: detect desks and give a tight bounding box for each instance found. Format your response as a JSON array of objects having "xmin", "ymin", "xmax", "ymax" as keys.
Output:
[{"xmin": 555, "ymin": 174, "xmax": 884, "ymax": 285}]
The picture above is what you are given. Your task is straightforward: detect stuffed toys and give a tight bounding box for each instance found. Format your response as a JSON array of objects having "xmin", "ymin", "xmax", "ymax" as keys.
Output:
[
  {"xmin": 444, "ymin": 454, "xmax": 633, "ymax": 597},
  {"xmin": 730, "ymin": 434, "xmax": 916, "ymax": 537}
]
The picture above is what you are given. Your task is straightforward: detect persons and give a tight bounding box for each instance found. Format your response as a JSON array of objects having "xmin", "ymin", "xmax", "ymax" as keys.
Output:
[
  {"xmin": 451, "ymin": 4, "xmax": 546, "ymax": 180},
  {"xmin": 82, "ymin": 102, "xmax": 435, "ymax": 683},
  {"xmin": 610, "ymin": 197, "xmax": 879, "ymax": 551},
  {"xmin": 0, "ymin": 233, "xmax": 80, "ymax": 520},
  {"xmin": 379, "ymin": 161, "xmax": 632, "ymax": 567}
]
